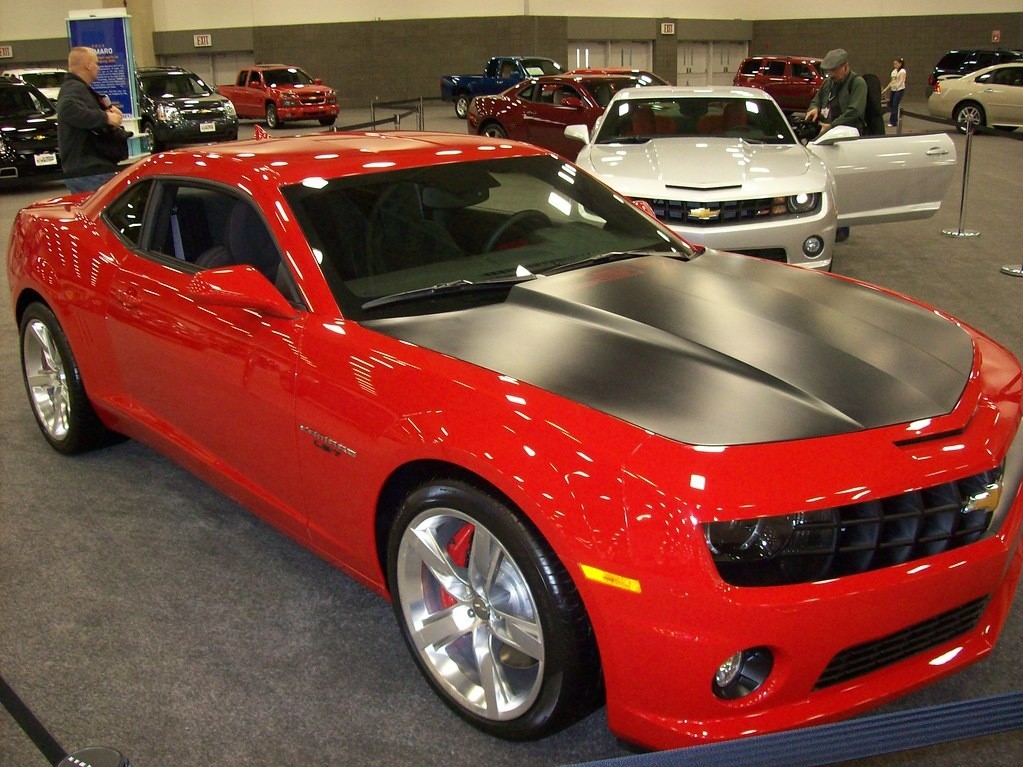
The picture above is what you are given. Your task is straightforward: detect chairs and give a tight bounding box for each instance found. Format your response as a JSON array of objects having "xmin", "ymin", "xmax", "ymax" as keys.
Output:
[
  {"xmin": 360, "ymin": 181, "xmax": 463, "ymax": 269},
  {"xmin": 553, "ymin": 90, "xmax": 565, "ymax": 104},
  {"xmin": 632, "ymin": 108, "xmax": 658, "ymax": 136},
  {"xmin": 502, "ymin": 64, "xmax": 514, "ymax": 78},
  {"xmin": 164, "ymin": 81, "xmax": 186, "ymax": 97},
  {"xmin": 597, "ymin": 86, "xmax": 611, "ymax": 106},
  {"xmin": 710, "ymin": 103, "xmax": 750, "ymax": 136},
  {"xmin": 224, "ymin": 193, "xmax": 343, "ymax": 307}
]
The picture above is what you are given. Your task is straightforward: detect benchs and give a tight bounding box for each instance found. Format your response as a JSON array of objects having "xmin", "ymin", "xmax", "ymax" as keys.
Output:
[{"xmin": 625, "ymin": 114, "xmax": 725, "ymax": 137}]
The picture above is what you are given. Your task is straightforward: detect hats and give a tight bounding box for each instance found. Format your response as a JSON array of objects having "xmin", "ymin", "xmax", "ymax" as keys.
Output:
[{"xmin": 819, "ymin": 48, "xmax": 848, "ymax": 69}]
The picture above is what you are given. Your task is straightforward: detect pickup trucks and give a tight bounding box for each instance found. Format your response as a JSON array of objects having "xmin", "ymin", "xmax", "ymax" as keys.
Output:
[
  {"xmin": 440, "ymin": 55, "xmax": 569, "ymax": 119},
  {"xmin": 212, "ymin": 63, "xmax": 341, "ymax": 129}
]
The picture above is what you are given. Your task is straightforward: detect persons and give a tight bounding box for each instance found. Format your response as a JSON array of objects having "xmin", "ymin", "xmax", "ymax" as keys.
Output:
[
  {"xmin": 882, "ymin": 57, "xmax": 907, "ymax": 128},
  {"xmin": 56, "ymin": 47, "xmax": 124, "ymax": 195},
  {"xmin": 804, "ymin": 49, "xmax": 868, "ymax": 242}
]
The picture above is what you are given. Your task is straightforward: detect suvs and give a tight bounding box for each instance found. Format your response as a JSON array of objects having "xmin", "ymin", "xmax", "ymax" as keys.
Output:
[
  {"xmin": 2, "ymin": 66, "xmax": 69, "ymax": 111},
  {"xmin": 928, "ymin": 48, "xmax": 1023, "ymax": 86},
  {"xmin": 0, "ymin": 74, "xmax": 64, "ymax": 190},
  {"xmin": 134, "ymin": 65, "xmax": 238, "ymax": 153},
  {"xmin": 734, "ymin": 54, "xmax": 828, "ymax": 115}
]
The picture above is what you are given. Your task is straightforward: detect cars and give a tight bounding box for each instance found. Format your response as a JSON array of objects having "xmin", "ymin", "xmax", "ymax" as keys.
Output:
[
  {"xmin": 927, "ymin": 62, "xmax": 1023, "ymax": 134},
  {"xmin": 465, "ymin": 74, "xmax": 655, "ymax": 165},
  {"xmin": 5, "ymin": 123, "xmax": 1023, "ymax": 752},
  {"xmin": 541, "ymin": 68, "xmax": 672, "ymax": 92},
  {"xmin": 561, "ymin": 85, "xmax": 958, "ymax": 273}
]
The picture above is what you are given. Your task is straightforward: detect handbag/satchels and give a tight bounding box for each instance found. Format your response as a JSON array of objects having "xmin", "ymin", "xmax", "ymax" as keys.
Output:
[{"xmin": 84, "ymin": 115, "xmax": 134, "ymax": 161}]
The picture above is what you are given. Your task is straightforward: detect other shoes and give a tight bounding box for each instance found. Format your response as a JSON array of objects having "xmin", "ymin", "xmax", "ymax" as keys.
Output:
[
  {"xmin": 835, "ymin": 227, "xmax": 849, "ymax": 242},
  {"xmin": 887, "ymin": 123, "xmax": 893, "ymax": 127}
]
